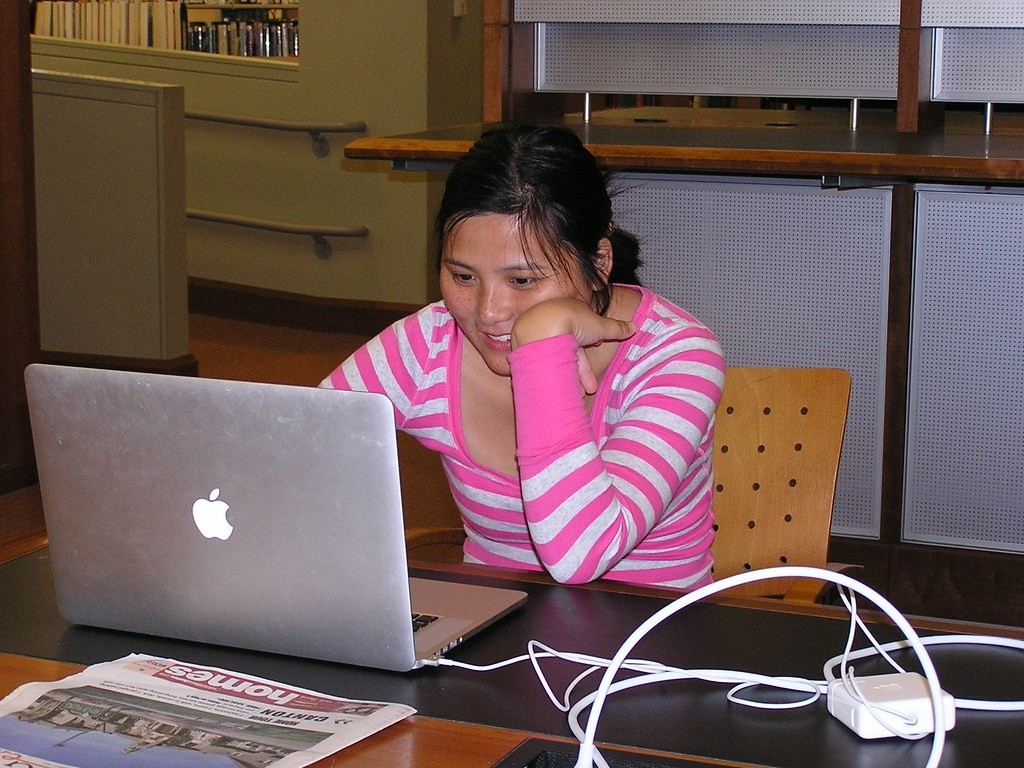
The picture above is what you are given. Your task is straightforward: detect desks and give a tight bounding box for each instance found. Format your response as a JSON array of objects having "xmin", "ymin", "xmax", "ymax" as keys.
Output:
[{"xmin": 1, "ymin": 94, "xmax": 1024, "ymax": 768}]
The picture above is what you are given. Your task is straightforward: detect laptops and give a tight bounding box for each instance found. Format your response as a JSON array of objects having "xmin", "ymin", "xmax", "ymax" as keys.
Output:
[{"xmin": 23, "ymin": 364, "xmax": 528, "ymax": 673}]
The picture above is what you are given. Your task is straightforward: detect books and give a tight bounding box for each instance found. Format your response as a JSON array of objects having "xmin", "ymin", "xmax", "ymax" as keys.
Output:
[{"xmin": 33, "ymin": 0, "xmax": 301, "ymax": 62}]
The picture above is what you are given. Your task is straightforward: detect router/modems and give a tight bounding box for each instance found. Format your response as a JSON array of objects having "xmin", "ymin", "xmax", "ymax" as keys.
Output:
[{"xmin": 826, "ymin": 672, "xmax": 955, "ymax": 739}]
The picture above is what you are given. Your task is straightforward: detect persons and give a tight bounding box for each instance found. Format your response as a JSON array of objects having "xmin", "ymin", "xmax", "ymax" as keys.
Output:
[{"xmin": 311, "ymin": 112, "xmax": 727, "ymax": 604}]
